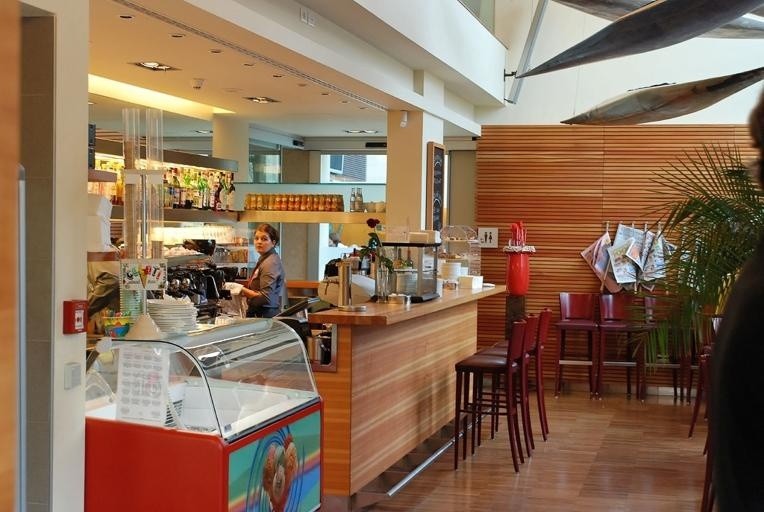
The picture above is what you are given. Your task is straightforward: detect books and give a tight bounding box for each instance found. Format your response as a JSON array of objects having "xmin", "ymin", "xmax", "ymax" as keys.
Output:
[{"xmin": 580, "ymin": 221, "xmax": 712, "ymax": 295}]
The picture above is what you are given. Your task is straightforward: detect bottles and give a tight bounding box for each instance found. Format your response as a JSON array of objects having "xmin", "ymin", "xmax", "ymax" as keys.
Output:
[
  {"xmin": 396, "ymin": 248, "xmax": 413, "ymax": 269},
  {"xmin": 349, "ymin": 188, "xmax": 363, "ymax": 212},
  {"xmin": 164, "ymin": 167, "xmax": 235, "ymax": 212}
]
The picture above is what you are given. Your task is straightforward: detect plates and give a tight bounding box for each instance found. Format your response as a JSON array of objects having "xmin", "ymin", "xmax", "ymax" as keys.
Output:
[
  {"xmin": 119, "ymin": 284, "xmax": 197, "ymax": 336},
  {"xmin": 441, "ymin": 263, "xmax": 468, "ymax": 282}
]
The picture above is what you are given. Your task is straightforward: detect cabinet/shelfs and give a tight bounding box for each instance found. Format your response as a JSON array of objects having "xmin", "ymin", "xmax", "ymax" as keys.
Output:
[{"xmin": 92, "ymin": 135, "xmax": 244, "ymax": 290}]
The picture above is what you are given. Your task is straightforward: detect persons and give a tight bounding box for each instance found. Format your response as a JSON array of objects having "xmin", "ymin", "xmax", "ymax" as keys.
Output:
[
  {"xmin": 241, "ymin": 223, "xmax": 285, "ymax": 318},
  {"xmin": 700, "ymin": 87, "xmax": 764, "ymax": 512}
]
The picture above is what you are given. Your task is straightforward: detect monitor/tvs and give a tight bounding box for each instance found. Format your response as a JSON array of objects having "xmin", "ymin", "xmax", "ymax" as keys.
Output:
[{"xmin": 272, "ymin": 297, "xmax": 321, "ymax": 317}]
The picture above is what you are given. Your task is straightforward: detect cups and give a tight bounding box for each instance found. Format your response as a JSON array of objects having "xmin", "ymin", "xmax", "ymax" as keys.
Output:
[{"xmin": 376, "ymin": 269, "xmax": 388, "ymax": 304}]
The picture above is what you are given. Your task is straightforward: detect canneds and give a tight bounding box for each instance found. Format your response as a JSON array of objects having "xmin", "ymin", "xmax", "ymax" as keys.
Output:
[{"xmin": 245, "ymin": 194, "xmax": 344, "ymax": 212}]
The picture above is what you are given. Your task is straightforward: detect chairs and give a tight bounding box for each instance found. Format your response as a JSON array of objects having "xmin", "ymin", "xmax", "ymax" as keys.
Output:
[
  {"xmin": 553, "ymin": 289, "xmax": 718, "ymax": 408},
  {"xmin": 451, "ymin": 307, "xmax": 551, "ymax": 473}
]
